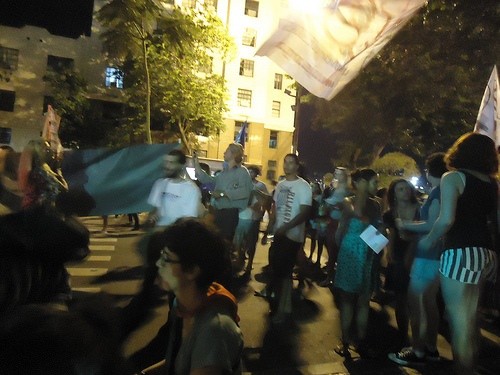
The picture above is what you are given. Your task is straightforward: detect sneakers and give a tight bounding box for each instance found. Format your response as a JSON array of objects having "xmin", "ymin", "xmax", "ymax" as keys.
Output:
[{"xmin": 387, "ymin": 346, "xmax": 428, "ymax": 365}]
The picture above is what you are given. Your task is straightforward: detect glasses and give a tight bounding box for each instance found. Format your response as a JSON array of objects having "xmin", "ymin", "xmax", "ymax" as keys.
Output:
[{"xmin": 160, "ymin": 250, "xmax": 183, "ymax": 267}]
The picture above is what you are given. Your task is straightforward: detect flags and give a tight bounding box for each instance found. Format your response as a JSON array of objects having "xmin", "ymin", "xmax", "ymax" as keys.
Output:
[
  {"xmin": 474, "ymin": 64, "xmax": 500, "ymax": 153},
  {"xmin": 42, "ymin": 105, "xmax": 73, "ymax": 153},
  {"xmin": 255, "ymin": 0, "xmax": 428, "ymax": 102},
  {"xmin": 55, "ymin": 143, "xmax": 184, "ymax": 216}
]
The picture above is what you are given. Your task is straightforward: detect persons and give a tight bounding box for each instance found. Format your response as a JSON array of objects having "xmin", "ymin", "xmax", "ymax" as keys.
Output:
[
  {"xmin": 100, "ymin": 211, "xmax": 140, "ymax": 233},
  {"xmin": 140, "ymin": 149, "xmax": 202, "ymax": 314},
  {"xmin": 0, "ymin": 145, "xmax": 25, "ymax": 219},
  {"xmin": 20, "ymin": 138, "xmax": 90, "ymax": 260},
  {"xmin": 420, "ymin": 132, "xmax": 500, "ymax": 374},
  {"xmin": 131, "ymin": 217, "xmax": 246, "ymax": 375},
  {"xmin": 182, "ymin": 125, "xmax": 454, "ymax": 369}
]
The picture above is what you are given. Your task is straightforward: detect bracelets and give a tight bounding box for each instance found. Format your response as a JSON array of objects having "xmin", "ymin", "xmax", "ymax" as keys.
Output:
[{"xmin": 137, "ymin": 370, "xmax": 148, "ymax": 375}]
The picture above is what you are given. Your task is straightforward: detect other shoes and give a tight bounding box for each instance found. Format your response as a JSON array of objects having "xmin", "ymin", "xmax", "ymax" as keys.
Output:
[
  {"xmin": 335, "ymin": 345, "xmax": 350, "ymax": 356},
  {"xmin": 254, "ymin": 289, "xmax": 273, "ymax": 299}
]
